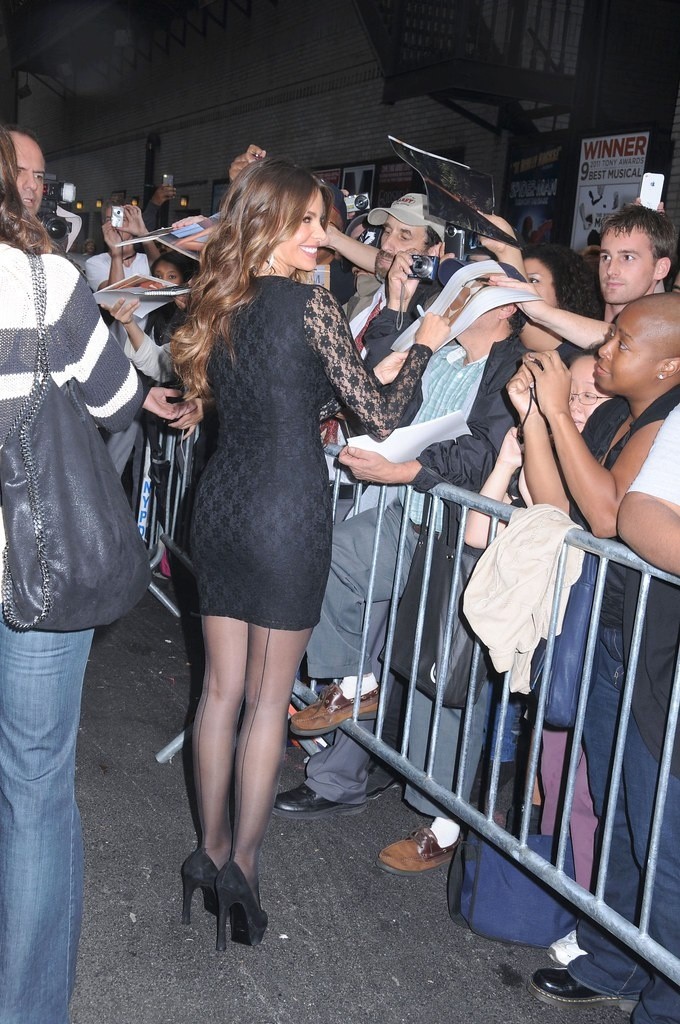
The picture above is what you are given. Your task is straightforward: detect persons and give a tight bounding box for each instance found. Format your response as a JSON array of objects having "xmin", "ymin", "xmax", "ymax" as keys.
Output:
[
  {"xmin": 85, "ymin": 142, "xmax": 680, "ymax": 1024},
  {"xmin": 0, "ymin": 130, "xmax": 145, "ymax": 1024},
  {"xmin": 3, "ymin": 126, "xmax": 45, "ymax": 214},
  {"xmin": 168, "ymin": 157, "xmax": 454, "ymax": 948}
]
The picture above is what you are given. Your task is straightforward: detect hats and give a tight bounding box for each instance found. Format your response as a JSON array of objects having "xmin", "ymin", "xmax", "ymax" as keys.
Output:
[
  {"xmin": 367, "ymin": 192, "xmax": 447, "ymax": 245},
  {"xmin": 437, "ymin": 257, "xmax": 527, "ymax": 302},
  {"xmin": 344, "ymin": 208, "xmax": 369, "ymax": 238}
]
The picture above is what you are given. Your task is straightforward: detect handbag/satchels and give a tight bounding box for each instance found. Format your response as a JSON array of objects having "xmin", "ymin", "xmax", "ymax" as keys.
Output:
[
  {"xmin": 378, "ymin": 467, "xmax": 501, "ymax": 708},
  {"xmin": 528, "ymin": 552, "xmax": 598, "ymax": 726},
  {"xmin": 445, "ymin": 819, "xmax": 580, "ymax": 948},
  {"xmin": 0, "ymin": 251, "xmax": 151, "ymax": 632}
]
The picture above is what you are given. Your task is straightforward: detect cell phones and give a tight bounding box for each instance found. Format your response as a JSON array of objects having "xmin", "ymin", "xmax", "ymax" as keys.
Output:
[
  {"xmin": 163, "ymin": 175, "xmax": 173, "ymax": 186},
  {"xmin": 639, "ymin": 173, "xmax": 665, "ymax": 212},
  {"xmin": 445, "ymin": 222, "xmax": 465, "ymax": 261}
]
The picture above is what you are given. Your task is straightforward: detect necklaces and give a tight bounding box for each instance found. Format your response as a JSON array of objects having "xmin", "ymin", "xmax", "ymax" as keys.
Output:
[{"xmin": 123, "ymin": 253, "xmax": 136, "ymax": 260}]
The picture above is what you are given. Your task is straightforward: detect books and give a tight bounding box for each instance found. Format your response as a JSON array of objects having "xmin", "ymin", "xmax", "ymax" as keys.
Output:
[
  {"xmin": 147, "ymin": 285, "xmax": 191, "ymax": 297},
  {"xmin": 116, "ymin": 234, "xmax": 199, "ymax": 260}
]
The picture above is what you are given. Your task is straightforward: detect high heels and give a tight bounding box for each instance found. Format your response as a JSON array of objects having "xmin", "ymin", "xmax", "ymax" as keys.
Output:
[
  {"xmin": 180, "ymin": 848, "xmax": 220, "ymax": 925},
  {"xmin": 215, "ymin": 860, "xmax": 267, "ymax": 951}
]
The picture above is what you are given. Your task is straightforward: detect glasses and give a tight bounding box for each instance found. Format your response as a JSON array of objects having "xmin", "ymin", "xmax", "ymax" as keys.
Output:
[{"xmin": 569, "ymin": 392, "xmax": 616, "ymax": 405}]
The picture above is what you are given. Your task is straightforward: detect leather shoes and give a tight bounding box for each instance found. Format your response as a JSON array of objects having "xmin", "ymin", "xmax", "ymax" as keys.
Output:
[
  {"xmin": 364, "ymin": 778, "xmax": 400, "ymax": 799},
  {"xmin": 290, "ymin": 682, "xmax": 379, "ymax": 736},
  {"xmin": 376, "ymin": 830, "xmax": 462, "ymax": 876},
  {"xmin": 271, "ymin": 782, "xmax": 367, "ymax": 819},
  {"xmin": 527, "ymin": 966, "xmax": 639, "ymax": 1012}
]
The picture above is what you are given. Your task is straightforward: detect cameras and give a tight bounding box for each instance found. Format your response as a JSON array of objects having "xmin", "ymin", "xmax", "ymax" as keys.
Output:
[
  {"xmin": 111, "ymin": 206, "xmax": 124, "ymax": 227},
  {"xmin": 408, "ymin": 255, "xmax": 440, "ymax": 281},
  {"xmin": 36, "ymin": 195, "xmax": 68, "ymax": 240},
  {"xmin": 344, "ymin": 193, "xmax": 370, "ymax": 212}
]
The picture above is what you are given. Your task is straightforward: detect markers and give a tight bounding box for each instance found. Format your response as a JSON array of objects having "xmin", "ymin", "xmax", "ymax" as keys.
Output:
[{"xmin": 415, "ymin": 305, "xmax": 426, "ymax": 320}]
